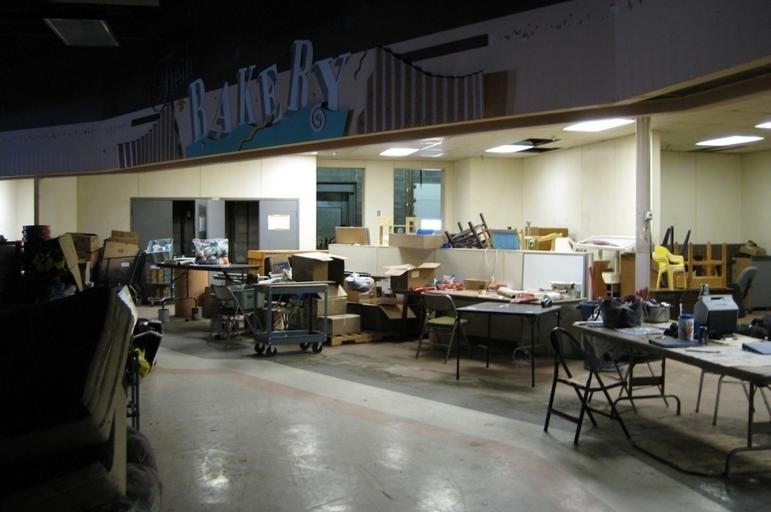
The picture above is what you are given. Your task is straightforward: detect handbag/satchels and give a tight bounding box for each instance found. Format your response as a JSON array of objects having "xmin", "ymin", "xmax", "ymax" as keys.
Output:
[
  {"xmin": 643, "ymin": 298, "xmax": 673, "ymax": 323},
  {"xmin": 590, "ymin": 293, "xmax": 643, "ymax": 329}
]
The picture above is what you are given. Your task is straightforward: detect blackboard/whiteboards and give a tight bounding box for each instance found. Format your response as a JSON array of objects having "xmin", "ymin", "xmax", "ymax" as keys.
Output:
[{"xmin": 522, "ymin": 252, "xmax": 586, "ymax": 298}]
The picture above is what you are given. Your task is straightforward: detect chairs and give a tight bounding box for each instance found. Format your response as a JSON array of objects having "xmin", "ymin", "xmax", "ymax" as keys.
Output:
[{"xmin": 206, "ymin": 283, "xmax": 264, "ymax": 350}]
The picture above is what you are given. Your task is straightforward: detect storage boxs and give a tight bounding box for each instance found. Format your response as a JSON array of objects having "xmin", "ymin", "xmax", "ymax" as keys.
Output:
[
  {"xmin": 290, "ymin": 248, "xmax": 440, "ymax": 337},
  {"xmin": 32, "ymin": 231, "xmax": 140, "ymax": 303}
]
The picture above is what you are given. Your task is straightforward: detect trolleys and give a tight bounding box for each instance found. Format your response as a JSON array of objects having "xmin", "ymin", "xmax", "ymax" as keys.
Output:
[{"xmin": 247, "ymin": 276, "xmax": 330, "ymax": 357}]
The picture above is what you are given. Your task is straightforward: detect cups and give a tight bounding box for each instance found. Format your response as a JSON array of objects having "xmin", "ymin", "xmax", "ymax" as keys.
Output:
[{"xmin": 677, "ymin": 314, "xmax": 695, "ymax": 341}]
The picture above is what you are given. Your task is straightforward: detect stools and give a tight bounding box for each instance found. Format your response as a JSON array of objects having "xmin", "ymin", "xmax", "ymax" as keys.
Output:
[{"xmin": 443, "ymin": 212, "xmax": 492, "ymax": 250}]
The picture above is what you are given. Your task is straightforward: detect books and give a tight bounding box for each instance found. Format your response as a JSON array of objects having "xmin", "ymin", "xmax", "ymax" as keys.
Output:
[
  {"xmin": 648, "ymin": 338, "xmax": 698, "ymax": 348},
  {"xmin": 740, "ymin": 341, "xmax": 770, "ymax": 355}
]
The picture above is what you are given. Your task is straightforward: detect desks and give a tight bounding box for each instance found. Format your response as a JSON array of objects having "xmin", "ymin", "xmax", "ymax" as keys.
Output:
[{"xmin": 155, "ymin": 263, "xmax": 262, "ymax": 340}]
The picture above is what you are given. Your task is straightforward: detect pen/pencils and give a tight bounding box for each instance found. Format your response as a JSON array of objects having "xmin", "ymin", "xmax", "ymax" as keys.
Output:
[{"xmin": 685, "ymin": 350, "xmax": 720, "ymax": 353}]
